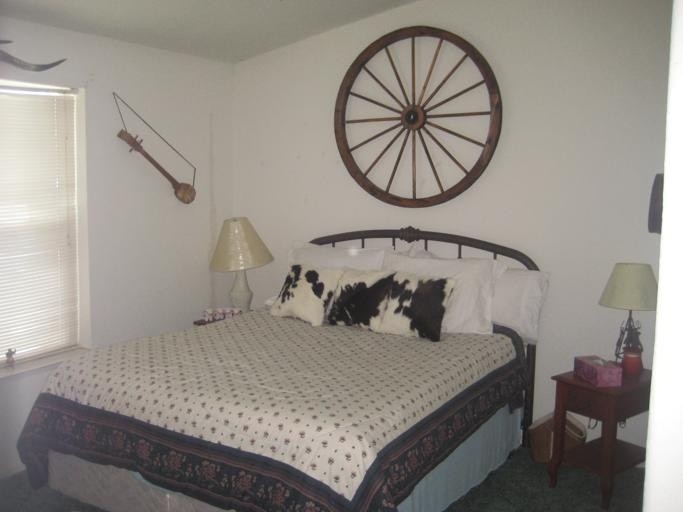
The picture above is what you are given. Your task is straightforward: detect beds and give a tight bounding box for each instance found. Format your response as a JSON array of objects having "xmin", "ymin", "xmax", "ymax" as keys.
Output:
[{"xmin": 17, "ymin": 227, "xmax": 540, "ymax": 512}]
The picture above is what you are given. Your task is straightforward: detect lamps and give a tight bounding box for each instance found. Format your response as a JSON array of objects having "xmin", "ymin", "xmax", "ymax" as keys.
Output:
[
  {"xmin": 598, "ymin": 262, "xmax": 658, "ymax": 364},
  {"xmin": 209, "ymin": 217, "xmax": 274, "ymax": 310}
]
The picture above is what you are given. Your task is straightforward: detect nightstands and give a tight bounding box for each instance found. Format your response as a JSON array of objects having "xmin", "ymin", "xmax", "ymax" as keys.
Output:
[{"xmin": 548, "ymin": 362, "xmax": 651, "ymax": 512}]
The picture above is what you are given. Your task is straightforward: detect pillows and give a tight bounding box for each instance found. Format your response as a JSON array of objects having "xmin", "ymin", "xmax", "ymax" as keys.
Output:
[{"xmin": 265, "ymin": 243, "xmax": 550, "ymax": 342}]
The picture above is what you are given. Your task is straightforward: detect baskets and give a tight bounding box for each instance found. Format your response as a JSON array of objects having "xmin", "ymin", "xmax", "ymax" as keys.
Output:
[{"xmin": 528, "ymin": 411, "xmax": 587, "ymax": 464}]
